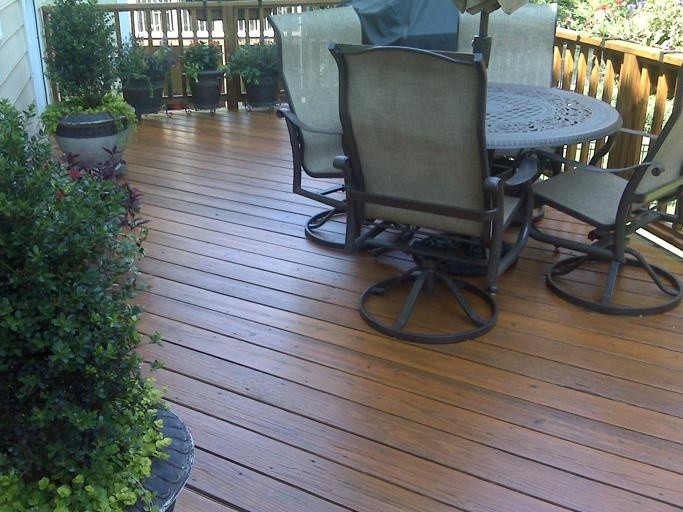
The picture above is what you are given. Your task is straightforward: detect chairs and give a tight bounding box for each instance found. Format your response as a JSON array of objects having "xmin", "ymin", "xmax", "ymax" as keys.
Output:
[
  {"xmin": 517, "ymin": 67, "xmax": 683, "ymax": 316},
  {"xmin": 327, "ymin": 43, "xmax": 537, "ymax": 345},
  {"xmin": 457, "ymin": 3, "xmax": 558, "ymax": 88},
  {"xmin": 269, "ymin": 5, "xmax": 407, "ymax": 251}
]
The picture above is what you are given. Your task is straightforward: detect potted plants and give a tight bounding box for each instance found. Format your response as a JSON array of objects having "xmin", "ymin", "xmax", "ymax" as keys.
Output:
[
  {"xmin": 39, "ymin": 90, "xmax": 139, "ymax": 180},
  {"xmin": 120, "ymin": 33, "xmax": 279, "ymax": 119}
]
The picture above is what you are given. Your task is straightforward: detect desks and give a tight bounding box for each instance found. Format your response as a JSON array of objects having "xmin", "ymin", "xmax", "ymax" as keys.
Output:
[{"xmin": 409, "ymin": 84, "xmax": 623, "ymax": 276}]
